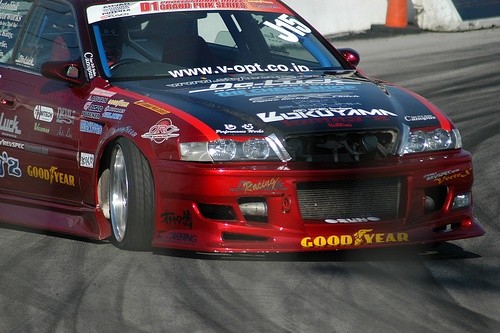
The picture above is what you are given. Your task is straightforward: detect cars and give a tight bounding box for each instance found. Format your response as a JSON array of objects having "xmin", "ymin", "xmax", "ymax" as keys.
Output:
[{"xmin": 0, "ymin": 0, "xmax": 487, "ymax": 253}]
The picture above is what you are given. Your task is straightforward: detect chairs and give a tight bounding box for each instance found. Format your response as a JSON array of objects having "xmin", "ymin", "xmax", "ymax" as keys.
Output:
[{"xmin": 120, "ymin": 28, "xmax": 163, "ymax": 64}]
